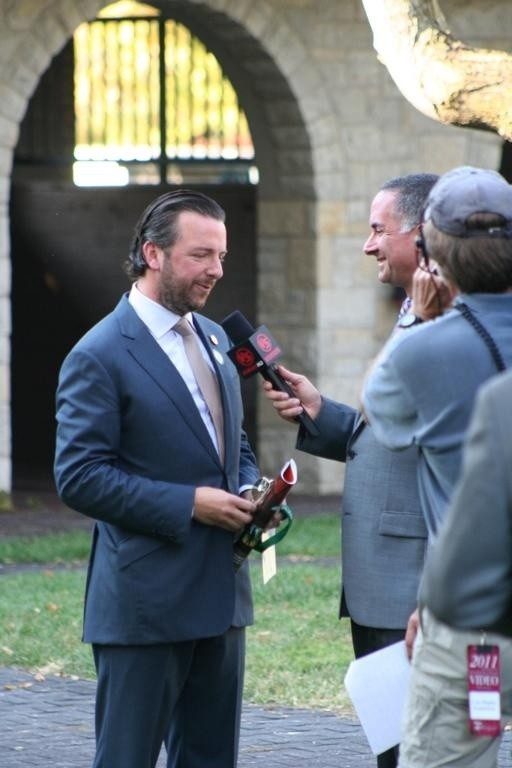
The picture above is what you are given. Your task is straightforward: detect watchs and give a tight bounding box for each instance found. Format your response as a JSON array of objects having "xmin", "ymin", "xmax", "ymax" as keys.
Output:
[{"xmin": 395, "ymin": 314, "xmax": 425, "ymax": 329}]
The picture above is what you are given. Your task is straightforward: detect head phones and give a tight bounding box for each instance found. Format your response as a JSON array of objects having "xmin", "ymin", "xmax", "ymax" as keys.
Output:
[{"xmin": 415, "ymin": 200, "xmax": 432, "ymax": 259}]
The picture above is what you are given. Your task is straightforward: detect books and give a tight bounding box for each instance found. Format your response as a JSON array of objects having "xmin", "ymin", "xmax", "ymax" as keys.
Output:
[{"xmin": 233, "ymin": 457, "xmax": 298, "ymax": 572}]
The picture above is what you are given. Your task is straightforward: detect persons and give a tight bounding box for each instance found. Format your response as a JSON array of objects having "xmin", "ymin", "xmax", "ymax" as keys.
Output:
[
  {"xmin": 397, "ymin": 370, "xmax": 511, "ymax": 767},
  {"xmin": 364, "ymin": 166, "xmax": 512, "ymax": 555},
  {"xmin": 260, "ymin": 173, "xmax": 463, "ymax": 768},
  {"xmin": 54, "ymin": 191, "xmax": 286, "ymax": 768}
]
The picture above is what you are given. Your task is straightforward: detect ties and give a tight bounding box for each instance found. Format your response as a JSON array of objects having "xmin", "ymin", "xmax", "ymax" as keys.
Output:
[{"xmin": 171, "ymin": 318, "xmax": 224, "ymax": 469}]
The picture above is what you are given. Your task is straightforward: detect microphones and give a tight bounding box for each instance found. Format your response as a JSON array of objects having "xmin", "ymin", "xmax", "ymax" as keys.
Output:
[
  {"xmin": 415, "ymin": 250, "xmax": 438, "ymax": 275},
  {"xmin": 220, "ymin": 310, "xmax": 320, "ymax": 440}
]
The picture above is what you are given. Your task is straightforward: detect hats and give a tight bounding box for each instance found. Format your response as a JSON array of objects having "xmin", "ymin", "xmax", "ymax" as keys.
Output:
[{"xmin": 419, "ymin": 165, "xmax": 512, "ymax": 237}]
